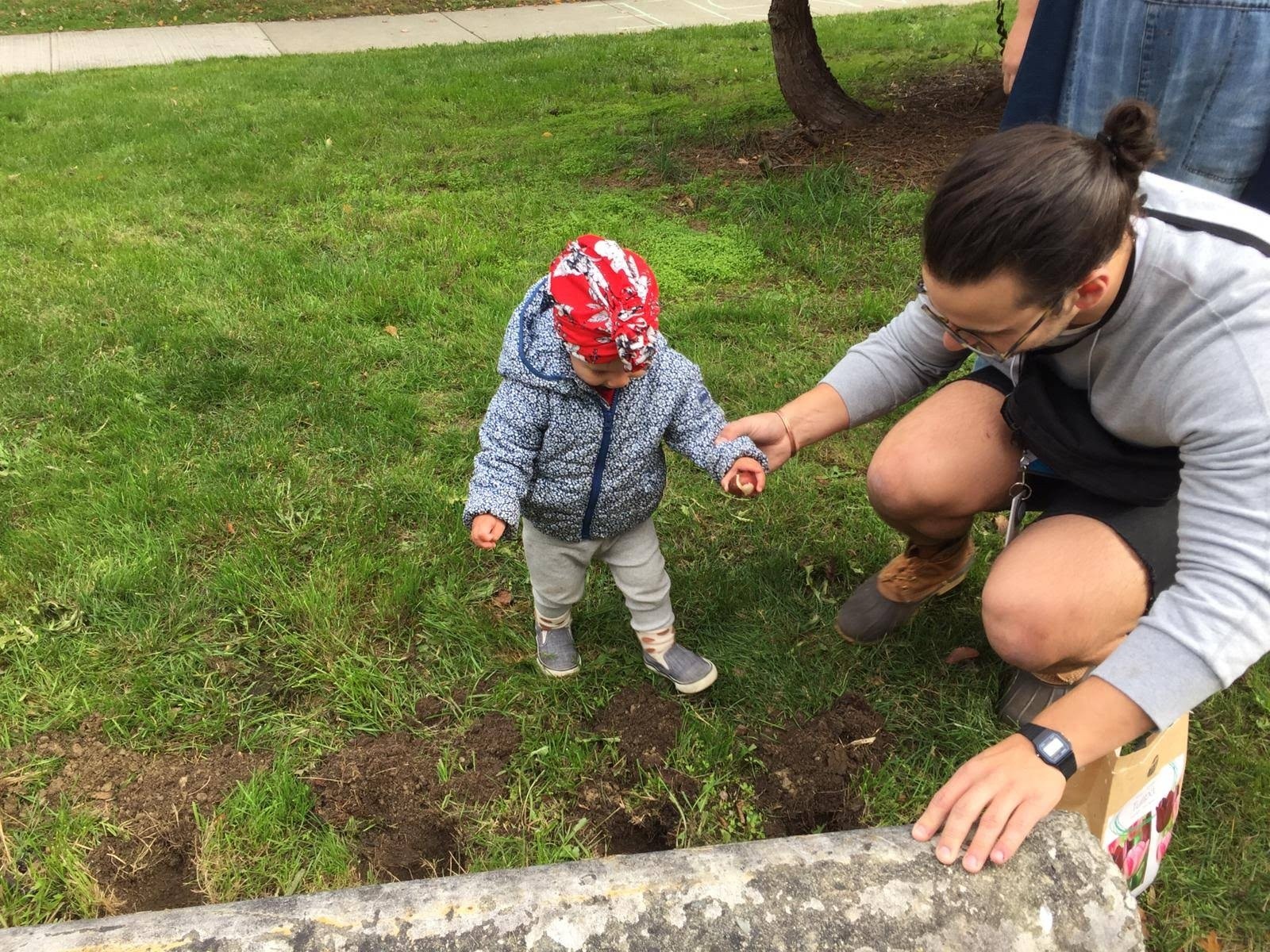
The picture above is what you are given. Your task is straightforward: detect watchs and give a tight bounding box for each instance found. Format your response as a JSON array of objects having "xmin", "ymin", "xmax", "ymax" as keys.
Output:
[{"xmin": 1017, "ymin": 720, "xmax": 1077, "ymax": 781}]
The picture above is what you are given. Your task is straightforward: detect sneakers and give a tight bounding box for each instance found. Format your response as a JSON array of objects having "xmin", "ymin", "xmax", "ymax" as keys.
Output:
[
  {"xmin": 534, "ymin": 618, "xmax": 581, "ymax": 677},
  {"xmin": 642, "ymin": 643, "xmax": 719, "ymax": 695}
]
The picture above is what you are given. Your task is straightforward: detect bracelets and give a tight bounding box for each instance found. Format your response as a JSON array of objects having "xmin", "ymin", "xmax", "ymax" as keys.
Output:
[{"xmin": 772, "ymin": 409, "xmax": 797, "ymax": 457}]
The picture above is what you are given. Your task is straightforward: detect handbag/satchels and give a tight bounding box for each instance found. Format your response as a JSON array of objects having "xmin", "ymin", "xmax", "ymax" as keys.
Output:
[{"xmin": 1046, "ymin": 708, "xmax": 1189, "ymax": 900}]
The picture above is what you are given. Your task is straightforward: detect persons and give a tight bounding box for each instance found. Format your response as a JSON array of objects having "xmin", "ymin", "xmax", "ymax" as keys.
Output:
[
  {"xmin": 999, "ymin": 0, "xmax": 1270, "ymax": 203},
  {"xmin": 717, "ymin": 96, "xmax": 1270, "ymax": 897},
  {"xmin": 460, "ymin": 234, "xmax": 769, "ymax": 694}
]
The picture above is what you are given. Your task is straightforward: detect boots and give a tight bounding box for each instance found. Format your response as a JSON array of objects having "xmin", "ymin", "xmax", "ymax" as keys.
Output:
[
  {"xmin": 996, "ymin": 656, "xmax": 1093, "ymax": 728},
  {"xmin": 836, "ymin": 517, "xmax": 974, "ymax": 646}
]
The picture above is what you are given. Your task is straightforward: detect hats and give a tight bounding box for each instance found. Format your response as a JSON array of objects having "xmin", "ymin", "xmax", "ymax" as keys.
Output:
[{"xmin": 549, "ymin": 234, "xmax": 660, "ymax": 373}]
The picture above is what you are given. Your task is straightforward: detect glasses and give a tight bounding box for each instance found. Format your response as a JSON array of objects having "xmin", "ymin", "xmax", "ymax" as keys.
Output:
[{"xmin": 912, "ymin": 282, "xmax": 1070, "ymax": 365}]
